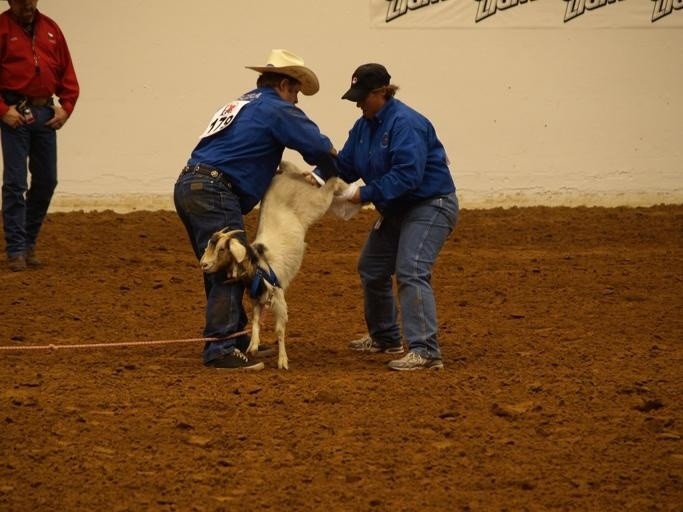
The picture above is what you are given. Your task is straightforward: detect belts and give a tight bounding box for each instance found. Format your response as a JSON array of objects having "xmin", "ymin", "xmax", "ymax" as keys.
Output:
[
  {"xmin": 181, "ymin": 162, "xmax": 240, "ymax": 197},
  {"xmin": 17, "ymin": 95, "xmax": 55, "ymax": 107}
]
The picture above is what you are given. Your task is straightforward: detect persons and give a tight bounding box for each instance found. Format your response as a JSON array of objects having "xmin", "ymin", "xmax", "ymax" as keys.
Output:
[
  {"xmin": 173, "ymin": 48, "xmax": 338, "ymax": 369},
  {"xmin": 0, "ymin": 0, "xmax": 82, "ymax": 272},
  {"xmin": 302, "ymin": 62, "xmax": 461, "ymax": 372}
]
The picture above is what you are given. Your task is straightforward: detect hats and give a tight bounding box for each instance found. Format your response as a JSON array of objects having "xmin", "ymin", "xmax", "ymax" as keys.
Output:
[
  {"xmin": 341, "ymin": 63, "xmax": 392, "ymax": 102},
  {"xmin": 243, "ymin": 48, "xmax": 320, "ymax": 96}
]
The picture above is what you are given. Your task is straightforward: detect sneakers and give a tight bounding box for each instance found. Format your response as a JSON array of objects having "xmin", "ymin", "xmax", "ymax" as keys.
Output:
[
  {"xmin": 243, "ymin": 342, "xmax": 272, "ymax": 358},
  {"xmin": 345, "ymin": 335, "xmax": 405, "ymax": 355},
  {"xmin": 202, "ymin": 348, "xmax": 265, "ymax": 373},
  {"xmin": 26, "ymin": 251, "xmax": 42, "ymax": 266},
  {"xmin": 387, "ymin": 351, "xmax": 444, "ymax": 372},
  {"xmin": 7, "ymin": 255, "xmax": 27, "ymax": 272}
]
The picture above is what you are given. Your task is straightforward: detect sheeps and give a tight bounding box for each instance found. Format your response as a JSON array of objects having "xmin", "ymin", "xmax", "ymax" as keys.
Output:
[{"xmin": 199, "ymin": 159, "xmax": 358, "ymax": 370}]
